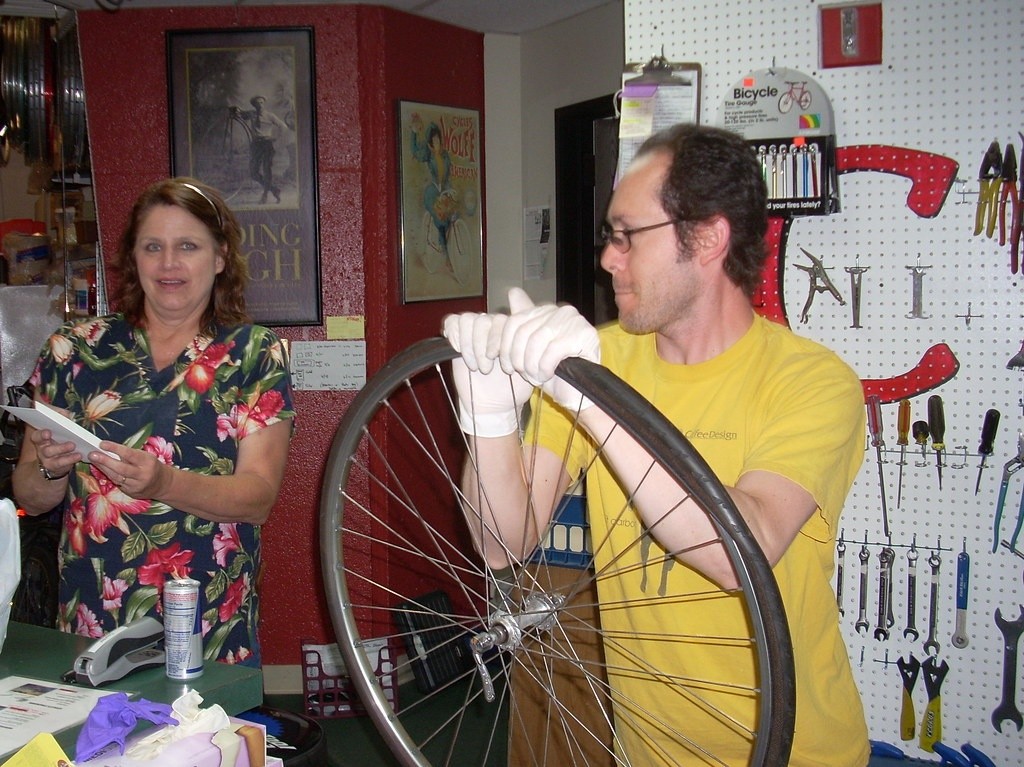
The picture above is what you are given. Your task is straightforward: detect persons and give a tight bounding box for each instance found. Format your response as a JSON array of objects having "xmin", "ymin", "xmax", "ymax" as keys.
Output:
[
  {"xmin": 230, "ymin": 94, "xmax": 287, "ymax": 203},
  {"xmin": 442, "ymin": 125, "xmax": 871, "ymax": 767},
  {"xmin": 0, "ymin": 176, "xmax": 296, "ymax": 669}
]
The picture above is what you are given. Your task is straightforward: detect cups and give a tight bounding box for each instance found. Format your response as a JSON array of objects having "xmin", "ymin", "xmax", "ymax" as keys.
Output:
[{"xmin": 3, "ymin": 232, "xmax": 52, "ymax": 286}]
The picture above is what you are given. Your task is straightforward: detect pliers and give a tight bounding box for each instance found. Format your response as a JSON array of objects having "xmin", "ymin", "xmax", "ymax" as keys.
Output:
[{"xmin": 974, "ymin": 141, "xmax": 1021, "ymax": 275}]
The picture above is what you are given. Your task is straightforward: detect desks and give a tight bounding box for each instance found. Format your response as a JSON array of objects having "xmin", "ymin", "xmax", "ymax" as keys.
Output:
[{"xmin": 0, "ymin": 621, "xmax": 262, "ymax": 767}]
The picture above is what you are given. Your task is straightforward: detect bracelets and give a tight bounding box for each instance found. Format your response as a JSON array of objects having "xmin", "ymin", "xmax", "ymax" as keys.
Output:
[{"xmin": 38, "ymin": 463, "xmax": 72, "ymax": 480}]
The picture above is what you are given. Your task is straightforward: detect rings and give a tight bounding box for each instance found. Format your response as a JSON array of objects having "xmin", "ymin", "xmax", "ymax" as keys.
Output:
[{"xmin": 122, "ymin": 477, "xmax": 125, "ymax": 483}]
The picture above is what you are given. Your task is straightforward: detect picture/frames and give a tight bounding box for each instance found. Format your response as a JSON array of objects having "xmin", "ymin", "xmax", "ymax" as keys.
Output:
[
  {"xmin": 163, "ymin": 24, "xmax": 325, "ymax": 326},
  {"xmin": 392, "ymin": 97, "xmax": 487, "ymax": 306}
]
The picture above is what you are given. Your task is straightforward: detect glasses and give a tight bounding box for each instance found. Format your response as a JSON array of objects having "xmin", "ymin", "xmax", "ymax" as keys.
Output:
[{"xmin": 601, "ymin": 217, "xmax": 684, "ymax": 253}]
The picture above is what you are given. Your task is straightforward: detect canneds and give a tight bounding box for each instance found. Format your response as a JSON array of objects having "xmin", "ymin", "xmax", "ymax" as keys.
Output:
[{"xmin": 163, "ymin": 579, "xmax": 204, "ymax": 680}]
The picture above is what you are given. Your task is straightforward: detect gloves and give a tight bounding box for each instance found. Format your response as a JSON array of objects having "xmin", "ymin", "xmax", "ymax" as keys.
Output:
[
  {"xmin": 498, "ymin": 304, "xmax": 601, "ymax": 411},
  {"xmin": 443, "ymin": 287, "xmax": 535, "ymax": 437}
]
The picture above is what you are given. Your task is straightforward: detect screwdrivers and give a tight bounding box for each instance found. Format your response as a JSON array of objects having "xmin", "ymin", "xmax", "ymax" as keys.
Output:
[
  {"xmin": 894, "ymin": 396, "xmax": 912, "ymax": 508},
  {"xmin": 973, "ymin": 408, "xmax": 1001, "ymax": 498},
  {"xmin": 865, "ymin": 394, "xmax": 893, "ymax": 537},
  {"xmin": 928, "ymin": 395, "xmax": 949, "ymax": 490}
]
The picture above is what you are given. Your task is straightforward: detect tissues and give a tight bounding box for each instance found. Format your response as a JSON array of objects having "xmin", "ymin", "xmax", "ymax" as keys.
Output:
[{"xmin": 71, "ymin": 687, "xmax": 268, "ymax": 767}]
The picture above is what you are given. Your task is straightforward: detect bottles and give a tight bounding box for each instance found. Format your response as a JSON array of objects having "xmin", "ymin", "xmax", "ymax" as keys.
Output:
[
  {"xmin": 71, "ymin": 269, "xmax": 96, "ymax": 317},
  {"xmin": 34, "ymin": 187, "xmax": 82, "ymax": 246}
]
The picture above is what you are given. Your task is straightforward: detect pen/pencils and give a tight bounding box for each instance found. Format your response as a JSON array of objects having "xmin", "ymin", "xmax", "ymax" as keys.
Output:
[{"xmin": 750, "ymin": 145, "xmax": 819, "ymax": 198}]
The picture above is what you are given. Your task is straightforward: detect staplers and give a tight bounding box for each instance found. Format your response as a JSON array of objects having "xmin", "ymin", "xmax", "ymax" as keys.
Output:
[{"xmin": 74, "ymin": 617, "xmax": 166, "ymax": 688}]
